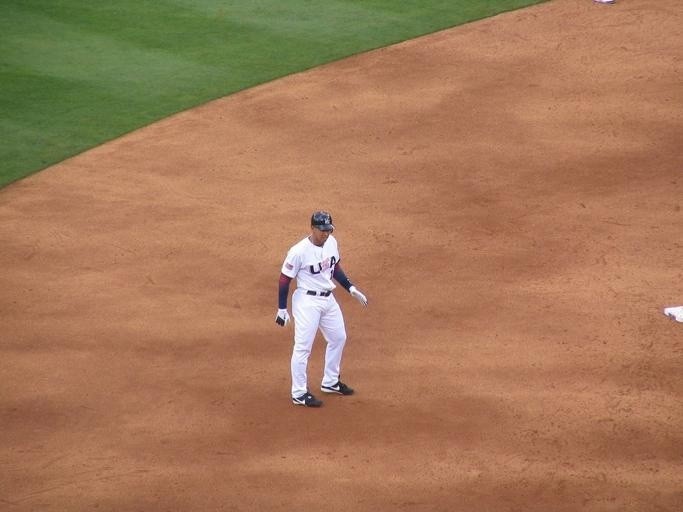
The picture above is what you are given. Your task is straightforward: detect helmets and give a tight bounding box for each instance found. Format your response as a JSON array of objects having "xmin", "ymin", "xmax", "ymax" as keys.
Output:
[{"xmin": 312, "ymin": 211, "xmax": 335, "ymax": 231}]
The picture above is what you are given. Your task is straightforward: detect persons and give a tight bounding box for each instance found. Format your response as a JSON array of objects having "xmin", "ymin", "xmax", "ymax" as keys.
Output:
[{"xmin": 275, "ymin": 211, "xmax": 368, "ymax": 408}]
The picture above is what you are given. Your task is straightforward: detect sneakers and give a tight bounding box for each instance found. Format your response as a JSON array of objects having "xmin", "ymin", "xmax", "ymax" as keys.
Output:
[
  {"xmin": 292, "ymin": 391, "xmax": 322, "ymax": 407},
  {"xmin": 321, "ymin": 381, "xmax": 353, "ymax": 395}
]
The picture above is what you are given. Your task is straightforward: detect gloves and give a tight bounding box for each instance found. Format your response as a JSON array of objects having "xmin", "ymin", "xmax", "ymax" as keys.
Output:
[
  {"xmin": 275, "ymin": 308, "xmax": 291, "ymax": 329},
  {"xmin": 349, "ymin": 286, "xmax": 368, "ymax": 307}
]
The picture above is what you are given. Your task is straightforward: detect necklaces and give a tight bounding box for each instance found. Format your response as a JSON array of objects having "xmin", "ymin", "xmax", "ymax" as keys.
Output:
[{"xmin": 310, "ymin": 234, "xmax": 324, "ymax": 260}]
